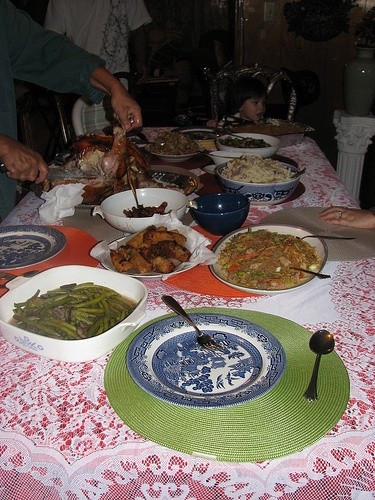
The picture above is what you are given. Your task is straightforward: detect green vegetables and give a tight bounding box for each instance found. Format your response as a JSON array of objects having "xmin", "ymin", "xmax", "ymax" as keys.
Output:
[{"xmin": 220, "ymin": 228, "xmax": 283, "ymax": 279}]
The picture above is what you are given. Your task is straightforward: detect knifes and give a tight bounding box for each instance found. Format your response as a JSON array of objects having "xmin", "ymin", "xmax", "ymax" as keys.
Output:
[{"xmin": 0, "ymin": 161, "xmax": 98, "ymax": 179}]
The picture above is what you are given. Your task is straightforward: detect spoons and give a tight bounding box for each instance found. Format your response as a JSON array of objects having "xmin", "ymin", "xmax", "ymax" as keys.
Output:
[{"xmin": 302, "ymin": 329, "xmax": 334, "ymax": 401}]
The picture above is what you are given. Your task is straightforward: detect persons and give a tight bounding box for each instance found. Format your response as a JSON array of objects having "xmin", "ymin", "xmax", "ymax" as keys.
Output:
[
  {"xmin": 0, "ymin": 0, "xmax": 143, "ymax": 222},
  {"xmin": 45, "ymin": 0, "xmax": 153, "ymax": 163},
  {"xmin": 318, "ymin": 206, "xmax": 375, "ymax": 229},
  {"xmin": 205, "ymin": 78, "xmax": 267, "ymax": 129}
]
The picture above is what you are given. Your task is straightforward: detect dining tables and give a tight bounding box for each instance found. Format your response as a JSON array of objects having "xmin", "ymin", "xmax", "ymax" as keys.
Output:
[{"xmin": 0, "ymin": 127, "xmax": 375, "ymax": 500}]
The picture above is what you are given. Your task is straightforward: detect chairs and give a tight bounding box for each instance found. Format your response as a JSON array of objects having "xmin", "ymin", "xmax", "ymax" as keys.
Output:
[{"xmin": 208, "ymin": 63, "xmax": 296, "ymax": 126}]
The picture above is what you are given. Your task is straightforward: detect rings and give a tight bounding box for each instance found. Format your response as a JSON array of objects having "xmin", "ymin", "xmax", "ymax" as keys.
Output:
[{"xmin": 339, "ymin": 211, "xmax": 342, "ymax": 218}]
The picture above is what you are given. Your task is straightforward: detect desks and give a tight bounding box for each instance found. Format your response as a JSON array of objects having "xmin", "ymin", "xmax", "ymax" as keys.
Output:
[{"xmin": 134, "ymin": 76, "xmax": 180, "ymax": 120}]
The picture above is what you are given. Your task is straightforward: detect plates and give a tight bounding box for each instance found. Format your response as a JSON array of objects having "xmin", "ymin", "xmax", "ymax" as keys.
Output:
[
  {"xmin": 0, "ymin": 225, "xmax": 67, "ymax": 271},
  {"xmin": 209, "ymin": 223, "xmax": 328, "ymax": 294},
  {"xmin": 125, "ymin": 313, "xmax": 286, "ymax": 409},
  {"xmin": 150, "ymin": 164, "xmax": 200, "ymax": 195},
  {"xmin": 171, "ymin": 126, "xmax": 222, "ymax": 141},
  {"xmin": 101, "ymin": 235, "xmax": 200, "ymax": 279}
]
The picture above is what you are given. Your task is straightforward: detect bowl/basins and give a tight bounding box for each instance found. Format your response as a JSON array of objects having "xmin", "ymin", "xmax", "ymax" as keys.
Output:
[
  {"xmin": 144, "ymin": 142, "xmax": 205, "ymax": 163},
  {"xmin": 215, "ymin": 160, "xmax": 300, "ymax": 205},
  {"xmin": 100, "ymin": 187, "xmax": 187, "ymax": 232},
  {"xmin": 208, "ymin": 150, "xmax": 242, "ymax": 166},
  {"xmin": 0, "ymin": 264, "xmax": 148, "ymax": 363},
  {"xmin": 233, "ymin": 119, "xmax": 315, "ymax": 147},
  {"xmin": 216, "ymin": 132, "xmax": 281, "ymax": 158},
  {"xmin": 189, "ymin": 193, "xmax": 250, "ymax": 233}
]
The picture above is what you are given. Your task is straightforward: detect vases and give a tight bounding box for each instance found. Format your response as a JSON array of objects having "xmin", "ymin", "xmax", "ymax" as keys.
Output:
[{"xmin": 342, "ymin": 43, "xmax": 375, "ymax": 116}]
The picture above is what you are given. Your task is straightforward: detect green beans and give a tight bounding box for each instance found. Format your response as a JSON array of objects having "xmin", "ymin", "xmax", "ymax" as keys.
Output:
[{"xmin": 13, "ymin": 283, "xmax": 136, "ymax": 340}]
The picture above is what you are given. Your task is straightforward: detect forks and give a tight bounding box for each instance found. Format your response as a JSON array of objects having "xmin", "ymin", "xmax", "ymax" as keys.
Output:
[{"xmin": 161, "ymin": 295, "xmax": 222, "ymax": 352}]
